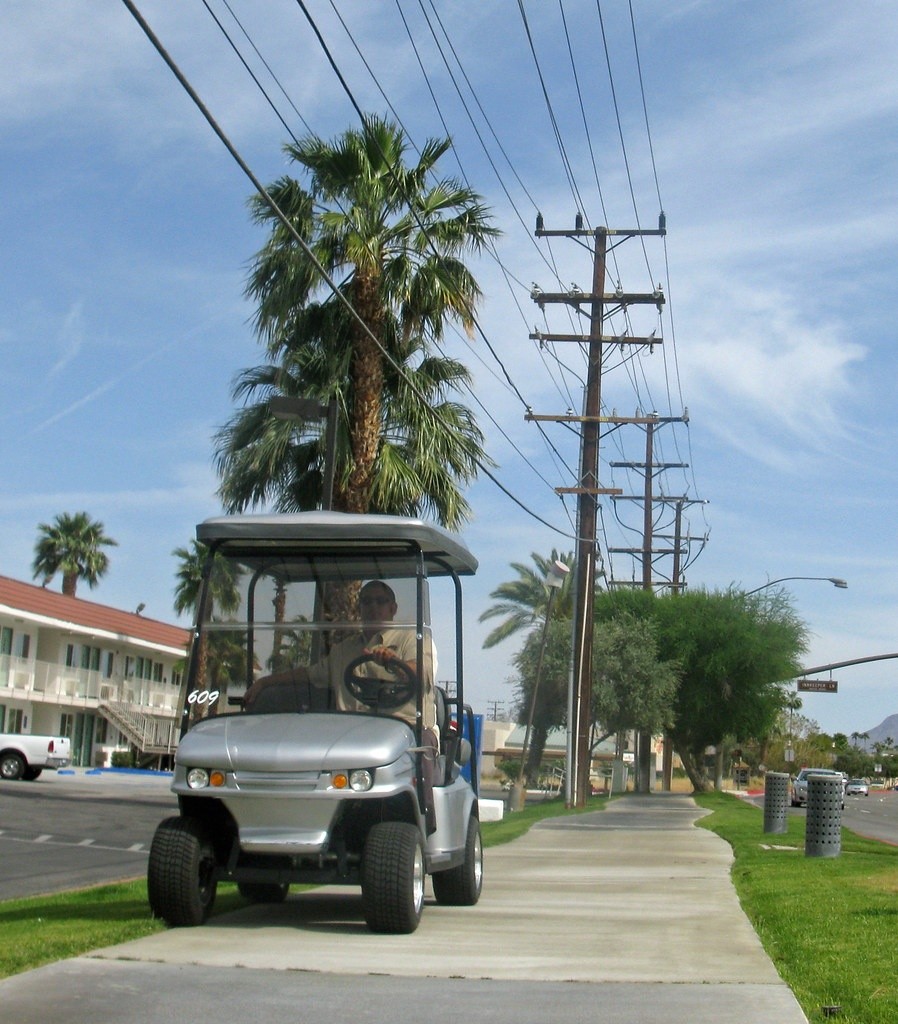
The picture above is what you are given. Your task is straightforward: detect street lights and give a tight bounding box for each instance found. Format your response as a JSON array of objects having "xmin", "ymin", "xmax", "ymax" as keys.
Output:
[{"xmin": 662, "ymin": 577, "xmax": 848, "ymax": 791}]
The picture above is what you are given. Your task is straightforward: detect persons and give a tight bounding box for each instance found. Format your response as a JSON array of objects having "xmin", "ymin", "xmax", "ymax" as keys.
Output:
[{"xmin": 243, "ymin": 581, "xmax": 441, "ymax": 839}]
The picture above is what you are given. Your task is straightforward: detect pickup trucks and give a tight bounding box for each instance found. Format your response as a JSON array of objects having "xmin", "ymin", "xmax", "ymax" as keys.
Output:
[{"xmin": 0, "ymin": 734, "xmax": 71, "ymax": 782}]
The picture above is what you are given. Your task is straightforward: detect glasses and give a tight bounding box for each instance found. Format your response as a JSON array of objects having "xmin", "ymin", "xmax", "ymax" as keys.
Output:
[{"xmin": 358, "ymin": 597, "xmax": 395, "ymax": 604}]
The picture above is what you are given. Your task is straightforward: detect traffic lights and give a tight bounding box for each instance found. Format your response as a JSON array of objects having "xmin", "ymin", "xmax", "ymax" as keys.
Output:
[{"xmin": 735, "ymin": 749, "xmax": 742, "ymax": 756}]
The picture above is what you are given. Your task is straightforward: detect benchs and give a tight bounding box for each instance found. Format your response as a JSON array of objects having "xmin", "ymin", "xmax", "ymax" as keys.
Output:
[{"xmin": 243, "ymin": 674, "xmax": 464, "ymax": 784}]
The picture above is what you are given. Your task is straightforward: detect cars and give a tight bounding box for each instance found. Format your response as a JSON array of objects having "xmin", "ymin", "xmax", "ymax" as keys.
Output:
[
  {"xmin": 870, "ymin": 780, "xmax": 884, "ymax": 788},
  {"xmin": 847, "ymin": 779, "xmax": 869, "ymax": 796},
  {"xmin": 791, "ymin": 768, "xmax": 845, "ymax": 810}
]
